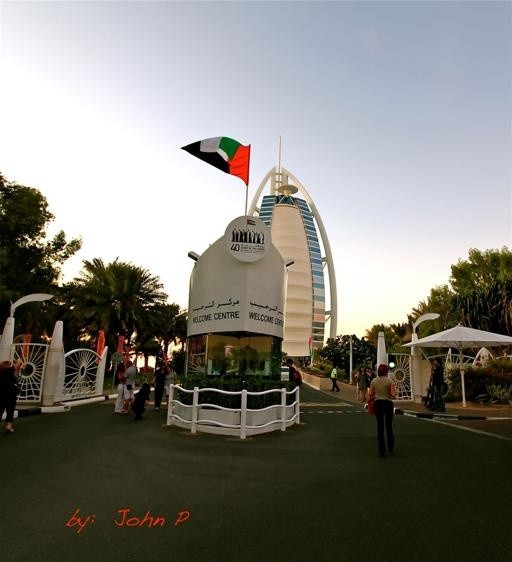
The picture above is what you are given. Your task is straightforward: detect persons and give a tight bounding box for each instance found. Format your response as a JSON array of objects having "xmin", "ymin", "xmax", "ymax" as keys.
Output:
[
  {"xmin": 153, "ymin": 362, "xmax": 167, "ymax": 411},
  {"xmin": 330, "ymin": 365, "xmax": 340, "ymax": 391},
  {"xmin": 352, "ymin": 365, "xmax": 376, "ymax": 402},
  {"xmin": 0, "ymin": 361, "xmax": 18, "ymax": 434},
  {"xmin": 165, "ymin": 366, "xmax": 178, "ymax": 403},
  {"xmin": 119, "ymin": 360, "xmax": 137, "ymax": 414},
  {"xmin": 431, "ymin": 358, "xmax": 445, "ymax": 404},
  {"xmin": 113, "ymin": 363, "xmax": 127, "ymax": 414},
  {"xmin": 368, "ymin": 364, "xmax": 397, "ymax": 458},
  {"xmin": 131, "ymin": 382, "xmax": 152, "ymax": 417},
  {"xmin": 284, "ymin": 358, "xmax": 300, "ymax": 387}
]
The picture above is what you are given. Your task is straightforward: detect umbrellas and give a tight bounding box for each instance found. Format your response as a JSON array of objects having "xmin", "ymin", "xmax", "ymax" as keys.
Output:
[{"xmin": 401, "ymin": 321, "xmax": 512, "ymax": 407}]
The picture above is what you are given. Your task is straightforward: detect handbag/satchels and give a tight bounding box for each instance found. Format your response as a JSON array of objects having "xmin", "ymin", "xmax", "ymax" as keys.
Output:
[{"xmin": 366, "ymin": 395, "xmax": 376, "ymax": 416}]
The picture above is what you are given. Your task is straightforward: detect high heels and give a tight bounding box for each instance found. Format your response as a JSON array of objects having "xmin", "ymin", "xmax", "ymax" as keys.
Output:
[{"xmin": 5, "ymin": 424, "xmax": 16, "ymax": 433}]
